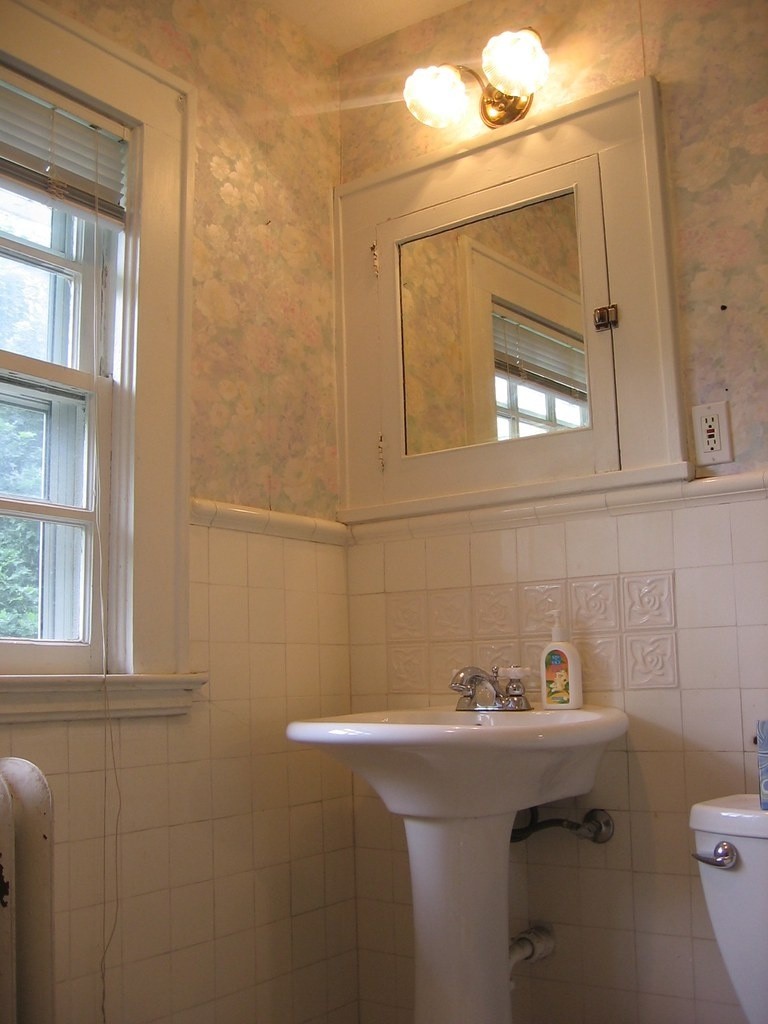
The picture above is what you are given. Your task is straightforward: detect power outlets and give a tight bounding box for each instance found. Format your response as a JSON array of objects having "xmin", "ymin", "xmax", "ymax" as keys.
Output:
[{"xmin": 691, "ymin": 400, "xmax": 734, "ymax": 468}]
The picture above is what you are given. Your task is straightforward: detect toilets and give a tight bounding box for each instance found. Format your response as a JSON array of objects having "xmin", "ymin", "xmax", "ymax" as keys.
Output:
[{"xmin": 688, "ymin": 792, "xmax": 767, "ymax": 1024}]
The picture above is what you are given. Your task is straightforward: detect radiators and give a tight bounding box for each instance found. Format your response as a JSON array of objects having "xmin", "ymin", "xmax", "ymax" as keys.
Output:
[{"xmin": 0, "ymin": 757, "xmax": 56, "ymax": 1024}]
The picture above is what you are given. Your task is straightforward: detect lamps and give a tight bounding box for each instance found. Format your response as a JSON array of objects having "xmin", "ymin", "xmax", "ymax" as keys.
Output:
[{"xmin": 402, "ymin": 27, "xmax": 550, "ymax": 130}]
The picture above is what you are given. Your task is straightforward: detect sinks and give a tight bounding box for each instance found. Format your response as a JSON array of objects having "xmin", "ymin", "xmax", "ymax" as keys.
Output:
[{"xmin": 285, "ymin": 701, "xmax": 631, "ymax": 821}]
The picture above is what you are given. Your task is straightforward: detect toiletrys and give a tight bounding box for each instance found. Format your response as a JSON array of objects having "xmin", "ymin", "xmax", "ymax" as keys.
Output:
[{"xmin": 539, "ymin": 609, "xmax": 584, "ymax": 711}]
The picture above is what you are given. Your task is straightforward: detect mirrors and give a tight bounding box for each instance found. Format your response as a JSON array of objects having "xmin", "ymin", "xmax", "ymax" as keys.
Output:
[{"xmin": 394, "ymin": 185, "xmax": 593, "ymax": 457}]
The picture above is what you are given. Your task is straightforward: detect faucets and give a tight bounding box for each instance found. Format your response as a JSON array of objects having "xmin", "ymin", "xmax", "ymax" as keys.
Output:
[{"xmin": 448, "ymin": 664, "xmax": 506, "ymax": 712}]
